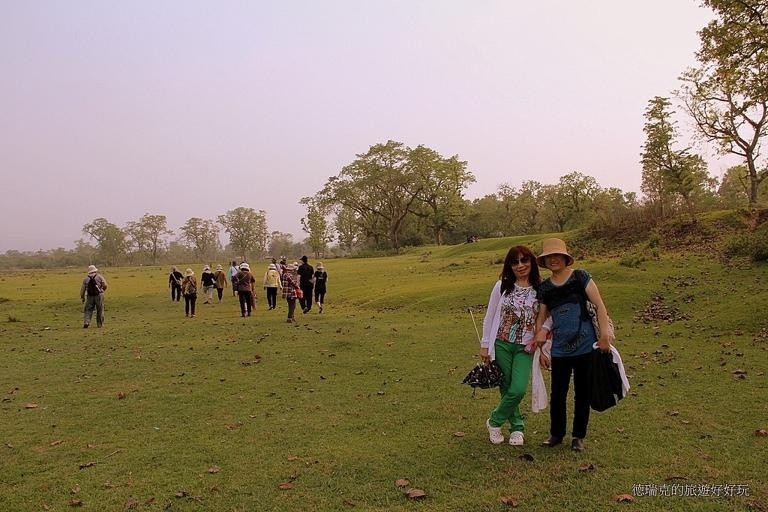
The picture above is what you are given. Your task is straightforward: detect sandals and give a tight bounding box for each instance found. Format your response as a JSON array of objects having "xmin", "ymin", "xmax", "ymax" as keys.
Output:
[
  {"xmin": 509, "ymin": 432, "xmax": 524, "ymax": 445},
  {"xmin": 486, "ymin": 418, "xmax": 504, "ymax": 444}
]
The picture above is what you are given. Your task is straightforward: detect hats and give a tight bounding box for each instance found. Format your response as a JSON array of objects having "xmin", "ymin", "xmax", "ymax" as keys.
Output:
[
  {"xmin": 88, "ymin": 265, "xmax": 98, "ymax": 273},
  {"xmin": 317, "ymin": 262, "xmax": 325, "ymax": 269},
  {"xmin": 185, "ymin": 269, "xmax": 194, "ymax": 276},
  {"xmin": 268, "ymin": 264, "xmax": 276, "ymax": 269},
  {"xmin": 204, "ymin": 265, "xmax": 223, "ymax": 270},
  {"xmin": 240, "ymin": 263, "xmax": 249, "ymax": 270},
  {"xmin": 286, "ymin": 263, "xmax": 297, "ymax": 270},
  {"xmin": 300, "ymin": 256, "xmax": 307, "ymax": 260},
  {"xmin": 537, "ymin": 239, "xmax": 574, "ymax": 267}
]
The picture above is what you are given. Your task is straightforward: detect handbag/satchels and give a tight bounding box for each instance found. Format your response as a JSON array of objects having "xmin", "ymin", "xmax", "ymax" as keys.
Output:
[
  {"xmin": 587, "ymin": 301, "xmax": 615, "ymax": 342},
  {"xmin": 177, "ymin": 280, "xmax": 182, "ymax": 285},
  {"xmin": 297, "ymin": 289, "xmax": 304, "ymax": 299}
]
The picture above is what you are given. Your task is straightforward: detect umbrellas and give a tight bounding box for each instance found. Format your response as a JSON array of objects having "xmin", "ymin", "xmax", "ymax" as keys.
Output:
[{"xmin": 462, "ymin": 307, "xmax": 505, "ymax": 389}]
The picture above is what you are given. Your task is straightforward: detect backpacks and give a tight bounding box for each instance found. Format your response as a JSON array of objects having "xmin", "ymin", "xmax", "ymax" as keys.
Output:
[{"xmin": 86, "ymin": 274, "xmax": 101, "ymax": 296}]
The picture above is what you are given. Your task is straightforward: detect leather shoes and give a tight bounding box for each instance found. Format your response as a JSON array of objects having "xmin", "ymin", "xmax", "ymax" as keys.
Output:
[
  {"xmin": 543, "ymin": 436, "xmax": 563, "ymax": 445},
  {"xmin": 572, "ymin": 439, "xmax": 584, "ymax": 451}
]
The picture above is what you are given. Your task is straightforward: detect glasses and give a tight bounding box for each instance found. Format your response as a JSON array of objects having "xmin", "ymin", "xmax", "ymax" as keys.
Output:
[{"xmin": 510, "ymin": 257, "xmax": 529, "ymax": 265}]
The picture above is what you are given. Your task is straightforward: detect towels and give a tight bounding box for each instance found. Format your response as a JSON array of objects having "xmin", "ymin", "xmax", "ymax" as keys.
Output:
[
  {"xmin": 593, "ymin": 340, "xmax": 631, "ymax": 398},
  {"xmin": 530, "ymin": 346, "xmax": 548, "ymax": 412}
]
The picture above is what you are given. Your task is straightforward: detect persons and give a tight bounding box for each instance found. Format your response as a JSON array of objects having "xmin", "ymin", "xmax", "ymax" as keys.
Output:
[
  {"xmin": 80, "ymin": 265, "xmax": 107, "ymax": 328},
  {"xmin": 536, "ymin": 238, "xmax": 610, "ymax": 451},
  {"xmin": 169, "ymin": 255, "xmax": 328, "ymax": 323},
  {"xmin": 479, "ymin": 245, "xmax": 553, "ymax": 446}
]
